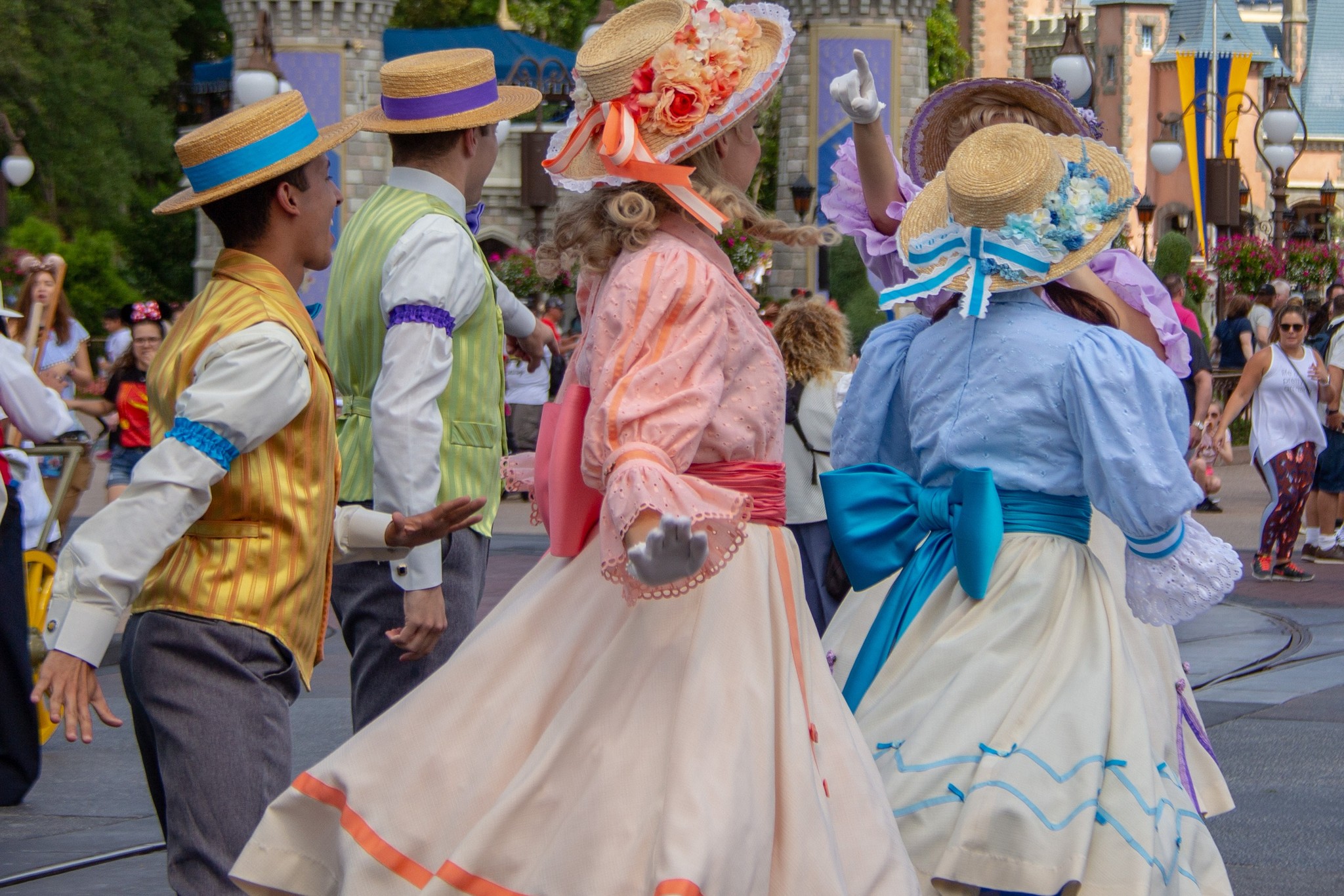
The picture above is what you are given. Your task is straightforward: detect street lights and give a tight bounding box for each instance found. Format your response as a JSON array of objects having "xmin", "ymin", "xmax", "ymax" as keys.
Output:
[
  {"xmin": 495, "ymin": 55, "xmax": 574, "ymax": 247},
  {"xmin": 1253, "ymin": 68, "xmax": 1308, "ymax": 279},
  {"xmin": 1149, "ymin": 91, "xmax": 1265, "ymax": 363},
  {"xmin": 1320, "ymin": 172, "xmax": 1338, "ymax": 249}
]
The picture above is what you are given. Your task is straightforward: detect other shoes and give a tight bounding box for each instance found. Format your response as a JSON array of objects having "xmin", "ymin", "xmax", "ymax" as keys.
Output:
[
  {"xmin": 1208, "ymin": 494, "xmax": 1220, "ymax": 504},
  {"xmin": 1195, "ymin": 496, "xmax": 1223, "ymax": 514},
  {"xmin": 1301, "ymin": 542, "xmax": 1344, "ymax": 564}
]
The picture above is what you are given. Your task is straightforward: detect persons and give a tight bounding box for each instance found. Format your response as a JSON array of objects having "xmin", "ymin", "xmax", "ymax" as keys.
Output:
[
  {"xmin": 768, "ymin": 297, "xmax": 857, "ymax": 641},
  {"xmin": 1163, "ymin": 274, "xmax": 1344, "ymax": 564},
  {"xmin": 228, "ymin": 0, "xmax": 933, "ymax": 896},
  {"xmin": 331, "ymin": 47, "xmax": 543, "ymax": 737},
  {"xmin": 501, "ymin": 291, "xmax": 582, "ymax": 502},
  {"xmin": 814, "ymin": 47, "xmax": 1241, "ymax": 896},
  {"xmin": 1212, "ymin": 303, "xmax": 1335, "ymax": 582},
  {"xmin": 0, "ymin": 252, "xmax": 189, "ymax": 807},
  {"xmin": 31, "ymin": 86, "xmax": 487, "ymax": 896}
]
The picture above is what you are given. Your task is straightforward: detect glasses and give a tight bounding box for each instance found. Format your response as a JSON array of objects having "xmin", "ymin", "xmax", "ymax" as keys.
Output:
[
  {"xmin": 1206, "ymin": 413, "xmax": 1218, "ymax": 419},
  {"xmin": 1279, "ymin": 323, "xmax": 1305, "ymax": 333}
]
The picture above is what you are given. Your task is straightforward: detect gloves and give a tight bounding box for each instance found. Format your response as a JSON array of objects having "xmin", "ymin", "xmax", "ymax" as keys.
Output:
[
  {"xmin": 625, "ymin": 515, "xmax": 709, "ymax": 586},
  {"xmin": 830, "ymin": 49, "xmax": 886, "ymax": 124}
]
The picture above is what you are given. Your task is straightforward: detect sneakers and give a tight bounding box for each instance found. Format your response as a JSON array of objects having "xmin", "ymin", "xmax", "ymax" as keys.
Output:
[
  {"xmin": 1271, "ymin": 561, "xmax": 1315, "ymax": 582},
  {"xmin": 1250, "ymin": 553, "xmax": 1273, "ymax": 582}
]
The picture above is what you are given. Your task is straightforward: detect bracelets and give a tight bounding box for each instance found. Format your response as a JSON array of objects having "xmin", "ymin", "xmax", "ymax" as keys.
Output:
[
  {"xmin": 67, "ymin": 365, "xmax": 77, "ymax": 376},
  {"xmin": 1319, "ymin": 374, "xmax": 1331, "ymax": 387}
]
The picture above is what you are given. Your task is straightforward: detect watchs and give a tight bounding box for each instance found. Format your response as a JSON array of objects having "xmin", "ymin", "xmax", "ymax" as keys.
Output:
[
  {"xmin": 1192, "ymin": 420, "xmax": 1204, "ymax": 431},
  {"xmin": 1325, "ymin": 408, "xmax": 1338, "ymax": 416}
]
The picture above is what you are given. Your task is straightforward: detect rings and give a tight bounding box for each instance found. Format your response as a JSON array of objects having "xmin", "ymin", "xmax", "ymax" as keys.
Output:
[{"xmin": 55, "ymin": 376, "xmax": 63, "ymax": 381}]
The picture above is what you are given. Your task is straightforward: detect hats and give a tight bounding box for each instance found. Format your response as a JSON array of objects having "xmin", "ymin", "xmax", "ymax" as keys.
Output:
[
  {"xmin": 879, "ymin": 123, "xmax": 1134, "ymax": 317},
  {"xmin": 1258, "ymin": 284, "xmax": 1276, "ymax": 296},
  {"xmin": 152, "ymin": 89, "xmax": 360, "ymax": 216},
  {"xmin": 344, "ymin": 47, "xmax": 543, "ymax": 134},
  {"xmin": 542, "ymin": 0, "xmax": 797, "ymax": 235},
  {"xmin": 902, "ymin": 77, "xmax": 1106, "ymax": 189}
]
